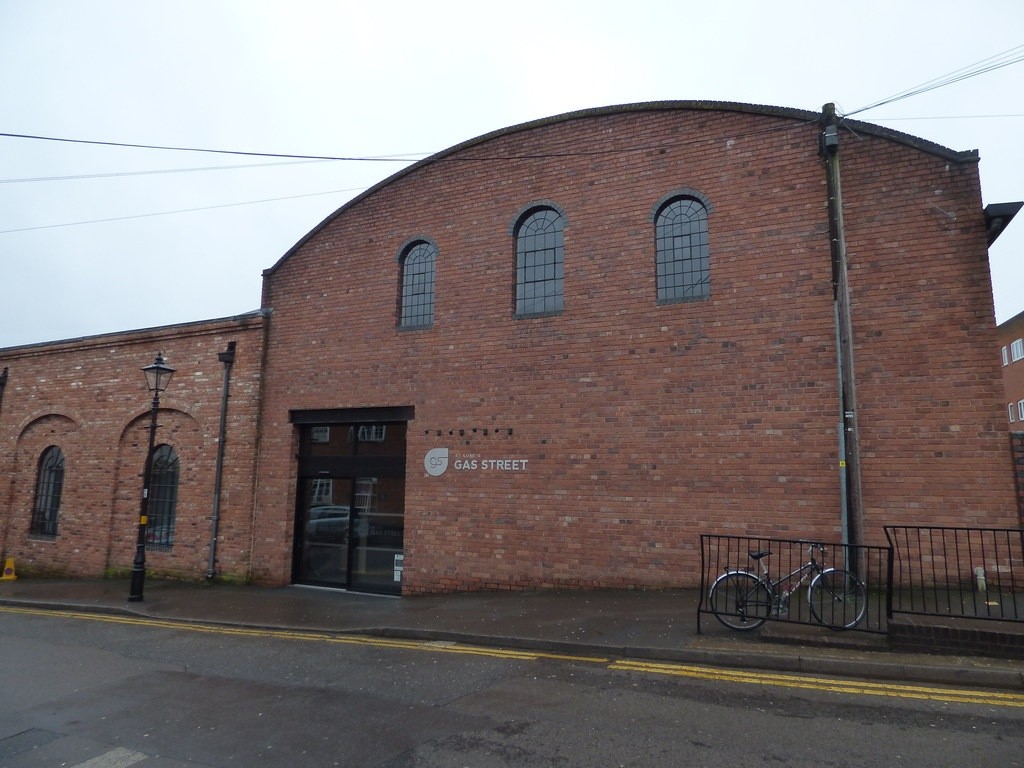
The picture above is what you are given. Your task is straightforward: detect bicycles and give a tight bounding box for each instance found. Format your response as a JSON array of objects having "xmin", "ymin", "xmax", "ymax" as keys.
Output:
[{"xmin": 711, "ymin": 538, "xmax": 872, "ymax": 633}]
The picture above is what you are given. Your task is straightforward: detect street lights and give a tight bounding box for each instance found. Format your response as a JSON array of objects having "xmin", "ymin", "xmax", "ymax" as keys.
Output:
[{"xmin": 124, "ymin": 350, "xmax": 178, "ymax": 604}]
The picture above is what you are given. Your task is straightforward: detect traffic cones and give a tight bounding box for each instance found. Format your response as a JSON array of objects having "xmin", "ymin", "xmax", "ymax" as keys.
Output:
[{"xmin": 0, "ymin": 556, "xmax": 17, "ymax": 580}]
